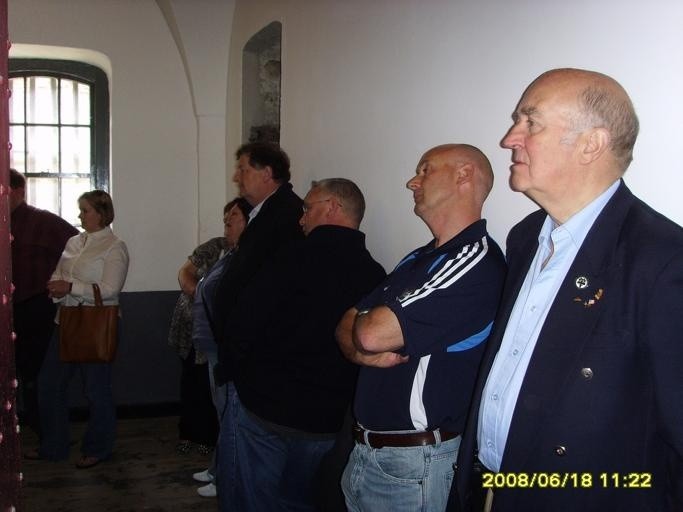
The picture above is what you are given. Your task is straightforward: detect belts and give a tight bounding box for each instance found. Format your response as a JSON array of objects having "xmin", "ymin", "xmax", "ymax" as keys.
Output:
[{"xmin": 351, "ymin": 426, "xmax": 471, "ymax": 448}]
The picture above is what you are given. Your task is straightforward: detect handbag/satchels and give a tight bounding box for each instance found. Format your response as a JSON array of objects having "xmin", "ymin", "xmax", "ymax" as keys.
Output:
[{"xmin": 56, "ymin": 280, "xmax": 120, "ymax": 367}]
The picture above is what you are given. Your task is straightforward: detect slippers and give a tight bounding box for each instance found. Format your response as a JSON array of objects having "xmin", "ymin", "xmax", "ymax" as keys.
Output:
[
  {"xmin": 76, "ymin": 454, "xmax": 102, "ymax": 468},
  {"xmin": 23, "ymin": 446, "xmax": 51, "ymax": 462}
]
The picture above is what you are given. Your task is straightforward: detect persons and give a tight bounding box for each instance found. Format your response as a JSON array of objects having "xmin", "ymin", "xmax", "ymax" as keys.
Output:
[
  {"xmin": 7, "ymin": 168, "xmax": 80, "ymax": 459},
  {"xmin": 221, "ymin": 198, "xmax": 239, "ymax": 224},
  {"xmin": 22, "ymin": 187, "xmax": 129, "ymax": 468},
  {"xmin": 194, "ymin": 143, "xmax": 305, "ymax": 500},
  {"xmin": 448, "ymin": 68, "xmax": 682, "ymax": 512},
  {"xmin": 154, "ymin": 198, "xmax": 250, "ymax": 454},
  {"xmin": 337, "ymin": 143, "xmax": 506, "ymax": 512},
  {"xmin": 218, "ymin": 177, "xmax": 390, "ymax": 512}
]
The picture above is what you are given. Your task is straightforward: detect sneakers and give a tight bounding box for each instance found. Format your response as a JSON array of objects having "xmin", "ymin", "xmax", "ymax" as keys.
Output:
[
  {"xmin": 191, "ymin": 468, "xmax": 218, "ymax": 481},
  {"xmin": 197, "ymin": 483, "xmax": 217, "ymax": 496}
]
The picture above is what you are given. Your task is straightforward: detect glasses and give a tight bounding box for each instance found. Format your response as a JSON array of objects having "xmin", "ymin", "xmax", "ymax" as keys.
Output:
[{"xmin": 300, "ymin": 200, "xmax": 342, "ymax": 211}]
